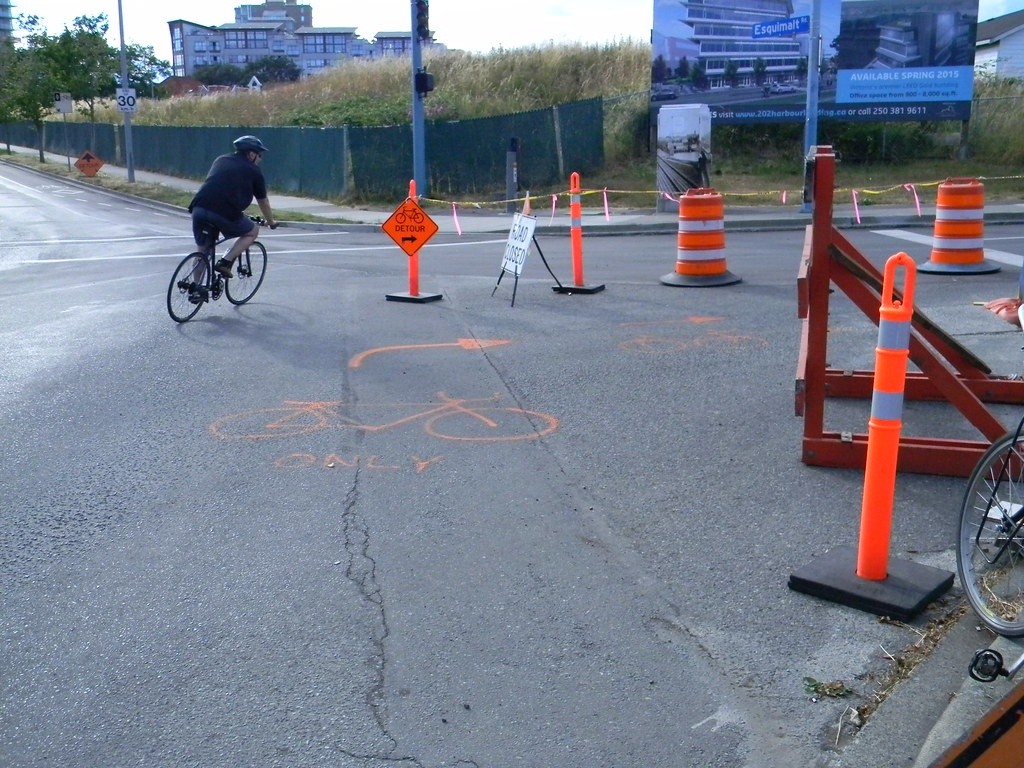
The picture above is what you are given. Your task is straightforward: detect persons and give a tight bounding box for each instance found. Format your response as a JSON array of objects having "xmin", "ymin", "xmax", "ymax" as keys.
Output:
[{"xmin": 188, "ymin": 135, "xmax": 279, "ymax": 303}]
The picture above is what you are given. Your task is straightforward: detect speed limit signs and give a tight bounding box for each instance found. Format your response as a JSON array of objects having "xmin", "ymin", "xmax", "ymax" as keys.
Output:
[{"xmin": 116, "ymin": 88, "xmax": 137, "ymax": 114}]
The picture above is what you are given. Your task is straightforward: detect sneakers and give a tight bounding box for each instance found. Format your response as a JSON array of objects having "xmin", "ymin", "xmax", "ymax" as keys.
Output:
[{"xmin": 214, "ymin": 260, "xmax": 233, "ymax": 279}]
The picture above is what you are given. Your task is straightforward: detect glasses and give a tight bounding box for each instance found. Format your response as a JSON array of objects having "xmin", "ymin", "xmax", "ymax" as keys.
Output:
[{"xmin": 257, "ymin": 153, "xmax": 262, "ymax": 158}]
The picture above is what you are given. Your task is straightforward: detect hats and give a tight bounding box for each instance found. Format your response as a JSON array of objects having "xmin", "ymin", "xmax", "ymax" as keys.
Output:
[{"xmin": 233, "ymin": 135, "xmax": 269, "ymax": 153}]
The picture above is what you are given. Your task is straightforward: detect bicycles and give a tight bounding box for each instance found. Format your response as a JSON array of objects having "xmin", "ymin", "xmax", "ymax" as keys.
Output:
[
  {"xmin": 955, "ymin": 302, "xmax": 1024, "ymax": 683},
  {"xmin": 167, "ymin": 211, "xmax": 278, "ymax": 324}
]
